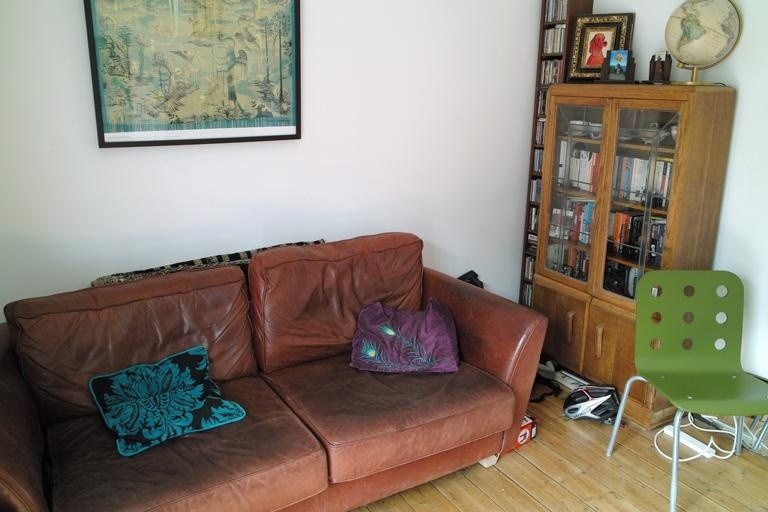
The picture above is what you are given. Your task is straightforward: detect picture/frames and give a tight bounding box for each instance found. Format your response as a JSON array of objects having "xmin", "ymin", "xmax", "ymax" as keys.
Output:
[
  {"xmin": 565, "ymin": 12, "xmax": 635, "ymax": 81},
  {"xmin": 82, "ymin": 0, "xmax": 304, "ymax": 148}
]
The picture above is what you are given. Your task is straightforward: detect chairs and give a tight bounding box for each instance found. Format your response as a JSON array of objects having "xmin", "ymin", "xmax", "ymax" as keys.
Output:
[{"xmin": 605, "ymin": 269, "xmax": 768, "ymax": 512}]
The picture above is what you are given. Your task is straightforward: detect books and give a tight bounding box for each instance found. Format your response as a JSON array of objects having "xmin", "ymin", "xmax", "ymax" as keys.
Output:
[
  {"xmin": 533, "ymin": 0, "xmax": 567, "ymax": 139},
  {"xmin": 520, "ymin": 138, "xmax": 670, "ymax": 306}
]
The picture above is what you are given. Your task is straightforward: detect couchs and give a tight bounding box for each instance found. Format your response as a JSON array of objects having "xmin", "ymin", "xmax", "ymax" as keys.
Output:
[{"xmin": 0, "ymin": 230, "xmax": 551, "ymax": 512}]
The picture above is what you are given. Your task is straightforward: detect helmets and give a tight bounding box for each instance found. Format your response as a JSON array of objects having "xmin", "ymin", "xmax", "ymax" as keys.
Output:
[{"xmin": 563, "ymin": 384, "xmax": 620, "ymax": 421}]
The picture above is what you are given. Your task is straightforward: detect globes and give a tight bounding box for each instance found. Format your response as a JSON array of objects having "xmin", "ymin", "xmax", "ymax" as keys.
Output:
[{"xmin": 665, "ymin": 0, "xmax": 744, "ymax": 86}]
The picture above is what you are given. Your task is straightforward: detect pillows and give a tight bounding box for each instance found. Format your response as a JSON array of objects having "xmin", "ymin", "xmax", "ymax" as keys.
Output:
[
  {"xmin": 86, "ymin": 345, "xmax": 246, "ymax": 458},
  {"xmin": 347, "ymin": 294, "xmax": 461, "ymax": 377}
]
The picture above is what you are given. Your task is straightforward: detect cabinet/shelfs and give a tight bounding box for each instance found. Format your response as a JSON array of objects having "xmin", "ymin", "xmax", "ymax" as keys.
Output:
[
  {"xmin": 531, "ymin": 83, "xmax": 737, "ymax": 433},
  {"xmin": 517, "ymin": 0, "xmax": 593, "ymax": 308}
]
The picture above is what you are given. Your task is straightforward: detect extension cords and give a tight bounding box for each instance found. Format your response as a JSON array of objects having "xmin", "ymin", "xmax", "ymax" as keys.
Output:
[{"xmin": 664, "ymin": 424, "xmax": 717, "ymax": 458}]
